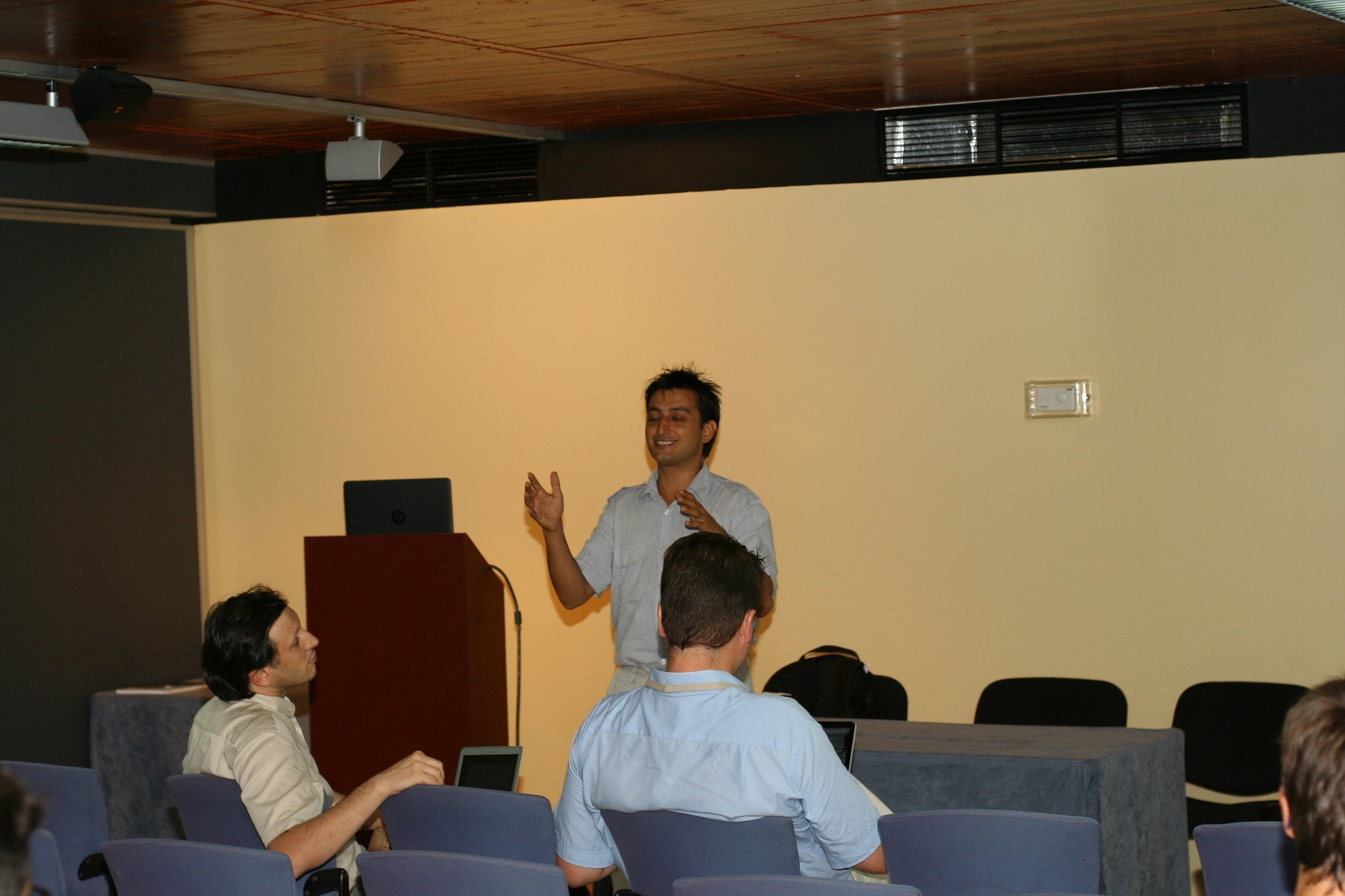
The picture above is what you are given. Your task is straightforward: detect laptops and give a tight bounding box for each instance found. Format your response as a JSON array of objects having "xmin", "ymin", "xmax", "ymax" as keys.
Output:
[
  {"xmin": 343, "ymin": 477, "xmax": 455, "ymax": 535},
  {"xmin": 813, "ymin": 720, "xmax": 857, "ymax": 773},
  {"xmin": 454, "ymin": 745, "xmax": 523, "ymax": 792}
]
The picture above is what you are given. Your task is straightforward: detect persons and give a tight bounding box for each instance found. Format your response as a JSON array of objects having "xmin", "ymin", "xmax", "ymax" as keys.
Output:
[
  {"xmin": 524, "ymin": 362, "xmax": 779, "ymax": 896},
  {"xmin": 561, "ymin": 530, "xmax": 903, "ymax": 885},
  {"xmin": 0, "ymin": 768, "xmax": 46, "ymax": 893},
  {"xmin": 178, "ymin": 584, "xmax": 447, "ymax": 896},
  {"xmin": 1278, "ymin": 675, "xmax": 1345, "ymax": 896}
]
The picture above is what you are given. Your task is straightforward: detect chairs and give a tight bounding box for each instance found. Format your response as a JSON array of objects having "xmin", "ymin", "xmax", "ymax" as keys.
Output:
[{"xmin": 0, "ymin": 653, "xmax": 1304, "ymax": 894}]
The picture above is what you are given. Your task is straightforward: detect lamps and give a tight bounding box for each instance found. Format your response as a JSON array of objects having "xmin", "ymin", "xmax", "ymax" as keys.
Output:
[
  {"xmin": 325, "ymin": 115, "xmax": 406, "ymax": 182},
  {"xmin": 71, "ymin": 63, "xmax": 151, "ymax": 117},
  {"xmin": 1, "ymin": 74, "xmax": 87, "ymax": 150}
]
